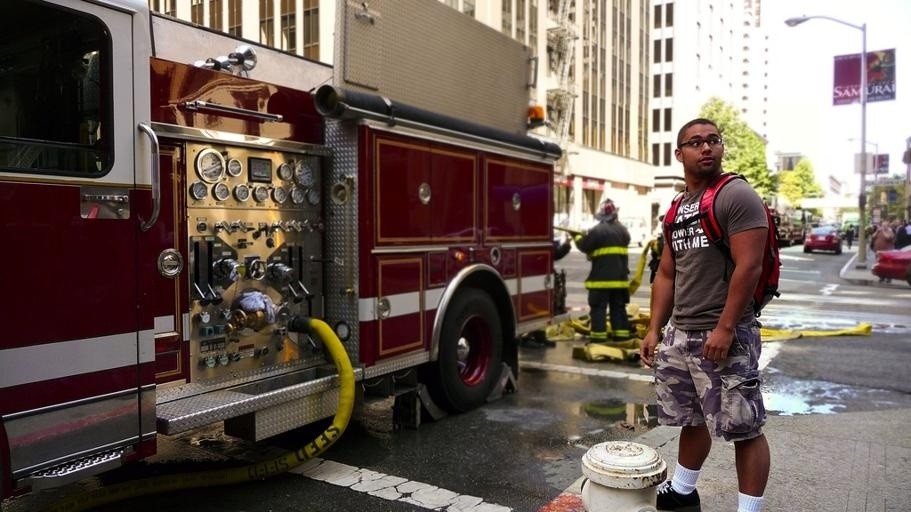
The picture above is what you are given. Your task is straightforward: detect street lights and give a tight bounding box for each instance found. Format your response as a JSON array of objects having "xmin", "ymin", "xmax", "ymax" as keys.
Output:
[{"xmin": 785, "ymin": 15, "xmax": 869, "ymax": 269}]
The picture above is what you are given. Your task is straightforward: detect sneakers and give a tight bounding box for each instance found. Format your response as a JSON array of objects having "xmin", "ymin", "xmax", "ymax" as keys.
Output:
[{"xmin": 656, "ymin": 481, "xmax": 701, "ymax": 510}]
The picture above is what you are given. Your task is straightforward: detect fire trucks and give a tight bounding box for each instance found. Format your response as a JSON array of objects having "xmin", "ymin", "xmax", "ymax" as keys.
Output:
[{"xmin": 0, "ymin": 2, "xmax": 562, "ymax": 503}]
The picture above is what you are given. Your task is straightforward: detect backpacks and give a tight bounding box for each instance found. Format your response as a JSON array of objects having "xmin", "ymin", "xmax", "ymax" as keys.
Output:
[{"xmin": 665, "ymin": 172, "xmax": 782, "ymax": 315}]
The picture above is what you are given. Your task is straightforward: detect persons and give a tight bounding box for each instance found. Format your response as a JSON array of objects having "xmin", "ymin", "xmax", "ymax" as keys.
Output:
[
  {"xmin": 553, "ymin": 231, "xmax": 572, "ymax": 261},
  {"xmin": 571, "ymin": 196, "xmax": 631, "ymax": 342},
  {"xmin": 870, "ymin": 218, "xmax": 911, "ymax": 283},
  {"xmin": 846, "ymin": 224, "xmax": 855, "ymax": 250},
  {"xmin": 646, "ymin": 214, "xmax": 665, "ymax": 286},
  {"xmin": 637, "ymin": 118, "xmax": 772, "ymax": 512}
]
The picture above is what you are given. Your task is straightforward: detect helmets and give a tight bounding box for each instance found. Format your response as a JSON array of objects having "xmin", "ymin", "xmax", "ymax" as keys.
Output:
[{"xmin": 595, "ymin": 199, "xmax": 620, "ymax": 222}]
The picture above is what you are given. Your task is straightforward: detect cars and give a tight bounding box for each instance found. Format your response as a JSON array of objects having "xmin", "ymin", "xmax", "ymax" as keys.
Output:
[
  {"xmin": 804, "ymin": 226, "xmax": 843, "ymax": 254},
  {"xmin": 872, "ymin": 244, "xmax": 911, "ymax": 287}
]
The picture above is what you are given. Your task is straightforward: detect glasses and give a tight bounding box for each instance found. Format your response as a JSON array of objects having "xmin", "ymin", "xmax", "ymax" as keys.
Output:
[{"xmin": 679, "ymin": 138, "xmax": 725, "ymax": 151}]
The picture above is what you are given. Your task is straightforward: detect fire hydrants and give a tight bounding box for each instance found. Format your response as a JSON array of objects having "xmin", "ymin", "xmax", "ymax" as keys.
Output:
[{"xmin": 579, "ymin": 440, "xmax": 667, "ymax": 512}]
